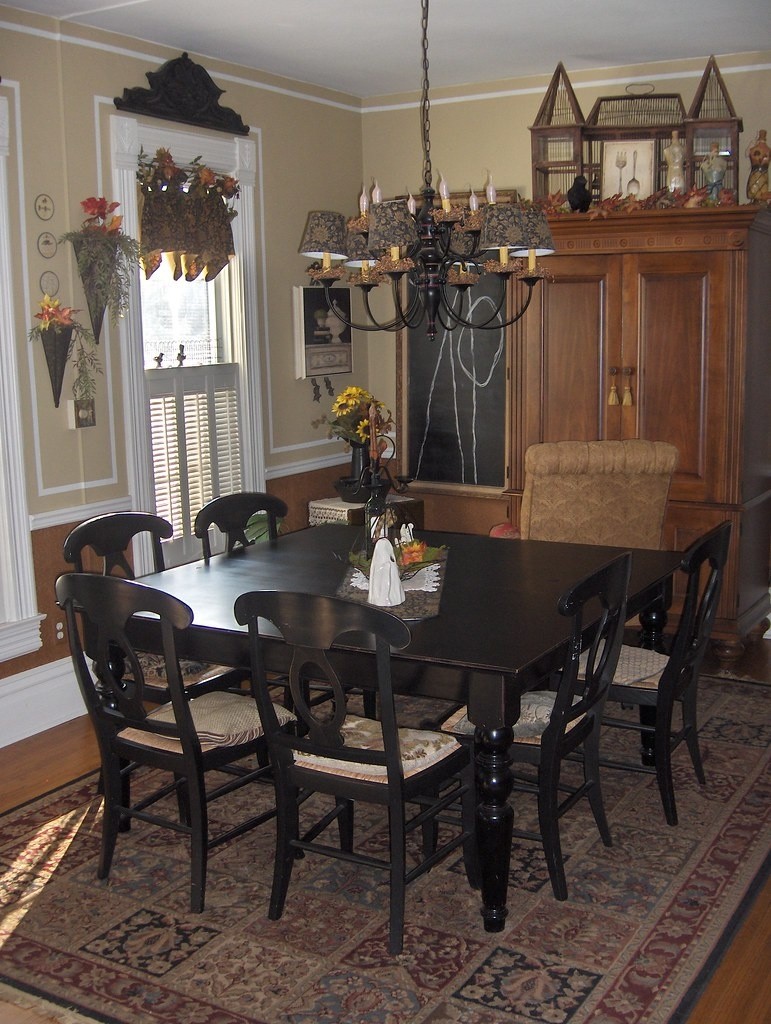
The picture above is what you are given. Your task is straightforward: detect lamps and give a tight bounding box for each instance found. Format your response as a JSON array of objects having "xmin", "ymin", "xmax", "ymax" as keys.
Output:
[{"xmin": 296, "ymin": 1, "xmax": 556, "ymax": 342}]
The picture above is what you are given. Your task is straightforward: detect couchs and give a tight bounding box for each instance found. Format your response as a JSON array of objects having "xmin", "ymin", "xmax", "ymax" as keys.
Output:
[{"xmin": 488, "ymin": 442, "xmax": 674, "ymax": 632}]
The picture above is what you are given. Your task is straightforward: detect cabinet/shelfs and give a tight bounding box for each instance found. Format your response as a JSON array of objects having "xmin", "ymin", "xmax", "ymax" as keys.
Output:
[{"xmin": 503, "ymin": 204, "xmax": 771, "ymax": 669}]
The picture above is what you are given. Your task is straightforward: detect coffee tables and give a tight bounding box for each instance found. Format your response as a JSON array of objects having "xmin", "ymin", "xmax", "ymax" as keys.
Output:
[{"xmin": 309, "ymin": 494, "xmax": 425, "ymax": 533}]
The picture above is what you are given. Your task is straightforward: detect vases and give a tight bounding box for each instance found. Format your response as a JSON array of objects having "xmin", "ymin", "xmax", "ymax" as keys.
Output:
[{"xmin": 351, "ymin": 445, "xmax": 371, "ymax": 484}]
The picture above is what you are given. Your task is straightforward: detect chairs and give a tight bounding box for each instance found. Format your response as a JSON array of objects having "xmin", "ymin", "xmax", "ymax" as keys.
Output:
[{"xmin": 51, "ymin": 493, "xmax": 732, "ymax": 952}]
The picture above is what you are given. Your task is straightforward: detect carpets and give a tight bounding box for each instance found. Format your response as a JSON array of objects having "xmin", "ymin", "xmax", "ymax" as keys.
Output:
[{"xmin": 1, "ymin": 650, "xmax": 770, "ymax": 1024}]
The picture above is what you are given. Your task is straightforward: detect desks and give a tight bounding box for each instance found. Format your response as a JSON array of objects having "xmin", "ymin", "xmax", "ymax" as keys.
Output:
[{"xmin": 56, "ymin": 527, "xmax": 693, "ymax": 932}]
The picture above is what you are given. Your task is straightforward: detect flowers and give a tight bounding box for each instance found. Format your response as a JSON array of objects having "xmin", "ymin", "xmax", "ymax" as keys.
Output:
[
  {"xmin": 308, "ymin": 386, "xmax": 397, "ymax": 462},
  {"xmin": 127, "ymin": 144, "xmax": 248, "ymax": 201},
  {"xmin": 57, "ymin": 196, "xmax": 145, "ymax": 328},
  {"xmin": 28, "ymin": 295, "xmax": 105, "ymax": 408}
]
